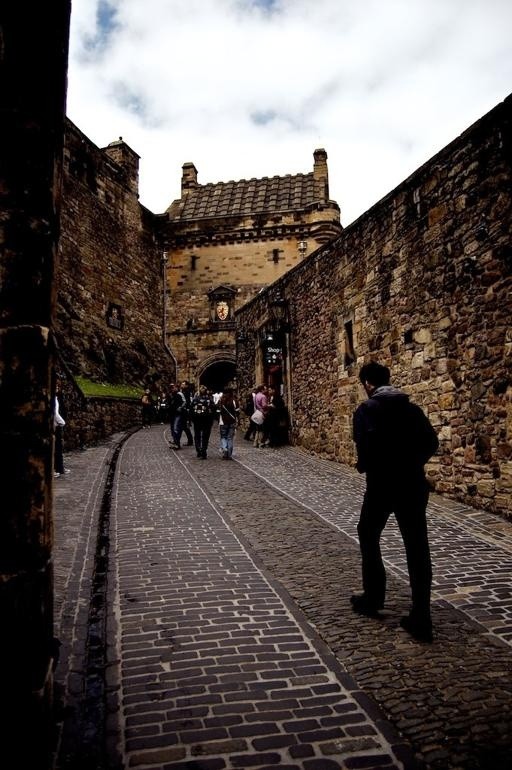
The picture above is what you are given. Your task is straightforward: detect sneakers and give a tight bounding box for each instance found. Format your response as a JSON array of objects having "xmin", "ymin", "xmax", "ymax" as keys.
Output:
[
  {"xmin": 402, "ymin": 616, "xmax": 431, "ymax": 640},
  {"xmin": 350, "ymin": 595, "xmax": 382, "ymax": 609},
  {"xmin": 53, "ymin": 469, "xmax": 69, "ymax": 477},
  {"xmin": 168, "ymin": 440, "xmax": 191, "ymax": 449},
  {"xmin": 194, "ymin": 449, "xmax": 232, "ymax": 459}
]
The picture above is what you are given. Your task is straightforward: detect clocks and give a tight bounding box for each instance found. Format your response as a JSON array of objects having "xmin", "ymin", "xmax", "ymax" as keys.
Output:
[{"xmin": 216, "ymin": 298, "xmax": 231, "ymax": 321}]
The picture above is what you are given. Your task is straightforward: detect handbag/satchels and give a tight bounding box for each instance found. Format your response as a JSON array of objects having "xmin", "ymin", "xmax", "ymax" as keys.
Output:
[{"xmin": 251, "ymin": 410, "xmax": 265, "ymax": 424}]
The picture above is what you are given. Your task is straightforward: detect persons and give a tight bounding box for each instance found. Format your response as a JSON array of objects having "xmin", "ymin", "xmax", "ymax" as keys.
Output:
[
  {"xmin": 350, "ymin": 363, "xmax": 439, "ymax": 644},
  {"xmin": 140, "ymin": 381, "xmax": 289, "ymax": 460},
  {"xmin": 51, "ymin": 378, "xmax": 65, "ymax": 478}
]
